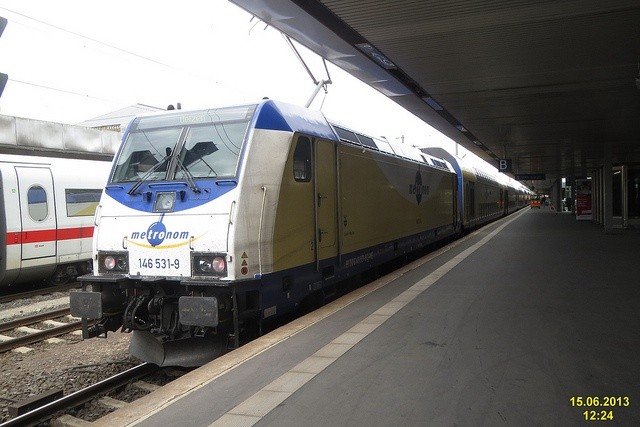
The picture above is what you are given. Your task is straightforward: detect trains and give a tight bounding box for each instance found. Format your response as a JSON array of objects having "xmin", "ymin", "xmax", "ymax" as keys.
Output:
[
  {"xmin": 70, "ymin": 98, "xmax": 533, "ymax": 366},
  {"xmin": 1, "ymin": 113, "xmax": 123, "ymax": 284}
]
